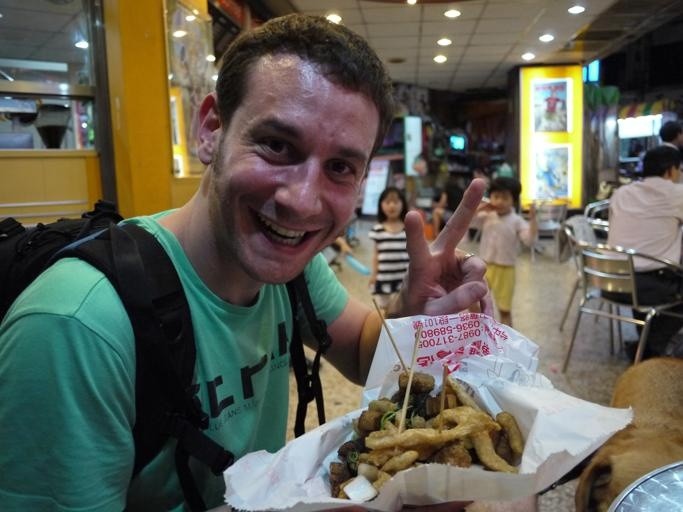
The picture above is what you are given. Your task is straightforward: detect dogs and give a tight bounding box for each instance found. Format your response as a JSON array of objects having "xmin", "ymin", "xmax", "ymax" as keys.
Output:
[{"xmin": 574, "ymin": 357, "xmax": 683, "ymax": 512}]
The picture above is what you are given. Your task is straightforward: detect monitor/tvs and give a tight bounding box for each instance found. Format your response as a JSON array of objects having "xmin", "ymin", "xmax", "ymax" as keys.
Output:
[
  {"xmin": 588, "ymin": 59, "xmax": 600, "ymax": 82},
  {"xmin": 449, "ymin": 133, "xmax": 467, "ymax": 154}
]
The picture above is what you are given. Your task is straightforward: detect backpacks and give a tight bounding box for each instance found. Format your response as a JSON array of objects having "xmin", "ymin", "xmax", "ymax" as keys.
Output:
[{"xmin": 0, "ymin": 199, "xmax": 333, "ymax": 512}]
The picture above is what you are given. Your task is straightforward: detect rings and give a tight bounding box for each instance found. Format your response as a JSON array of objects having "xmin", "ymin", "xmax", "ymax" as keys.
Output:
[{"xmin": 462, "ymin": 252, "xmax": 475, "ymax": 264}]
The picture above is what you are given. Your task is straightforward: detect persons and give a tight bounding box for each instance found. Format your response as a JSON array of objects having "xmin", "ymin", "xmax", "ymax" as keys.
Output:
[
  {"xmin": 656, "ymin": 121, "xmax": 683, "ymax": 183},
  {"xmin": 598, "ymin": 146, "xmax": 683, "ymax": 361},
  {"xmin": 542, "ymin": 90, "xmax": 561, "ymax": 121},
  {"xmin": 320, "ymin": 234, "xmax": 353, "ymax": 275},
  {"xmin": 627, "ymin": 139, "xmax": 642, "ymax": 167},
  {"xmin": 1, "ymin": 11, "xmax": 487, "ymax": 512},
  {"xmin": 367, "ymin": 156, "xmax": 542, "ymax": 328}
]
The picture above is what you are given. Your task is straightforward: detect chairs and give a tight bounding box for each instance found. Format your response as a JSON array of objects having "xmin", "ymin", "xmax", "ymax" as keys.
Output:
[{"xmin": 555, "ymin": 198, "xmax": 683, "ymax": 374}]
